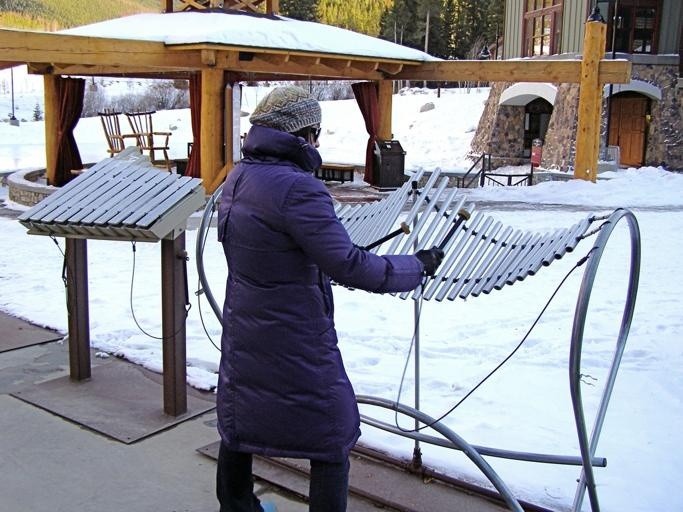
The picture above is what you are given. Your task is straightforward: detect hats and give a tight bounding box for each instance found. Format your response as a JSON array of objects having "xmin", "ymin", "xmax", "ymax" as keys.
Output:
[{"xmin": 249, "ymin": 85, "xmax": 322, "ymax": 134}]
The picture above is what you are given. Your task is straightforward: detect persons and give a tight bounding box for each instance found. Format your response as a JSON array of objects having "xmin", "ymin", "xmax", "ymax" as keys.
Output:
[{"xmin": 217, "ymin": 85, "xmax": 444, "ymax": 512}]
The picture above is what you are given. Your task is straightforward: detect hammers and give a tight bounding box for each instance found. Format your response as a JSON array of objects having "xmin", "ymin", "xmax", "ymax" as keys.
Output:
[
  {"xmin": 438, "ymin": 209, "xmax": 470, "ymax": 251},
  {"xmin": 367, "ymin": 222, "xmax": 409, "ymax": 251}
]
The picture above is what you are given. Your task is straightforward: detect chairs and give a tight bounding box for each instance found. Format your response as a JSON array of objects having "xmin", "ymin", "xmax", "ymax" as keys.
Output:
[{"xmin": 97, "ymin": 106, "xmax": 171, "ymax": 174}]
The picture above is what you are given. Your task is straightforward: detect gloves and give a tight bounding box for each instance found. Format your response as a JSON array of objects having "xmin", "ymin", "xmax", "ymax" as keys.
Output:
[{"xmin": 416, "ymin": 245, "xmax": 445, "ymax": 278}]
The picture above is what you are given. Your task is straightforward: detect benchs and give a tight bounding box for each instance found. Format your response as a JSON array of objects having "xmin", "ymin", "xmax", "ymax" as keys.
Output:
[{"xmin": 315, "ymin": 163, "xmax": 354, "ymax": 185}]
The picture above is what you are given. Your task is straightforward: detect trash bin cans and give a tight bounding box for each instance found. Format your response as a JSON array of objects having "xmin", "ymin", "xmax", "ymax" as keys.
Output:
[
  {"xmin": 531, "ymin": 137, "xmax": 542, "ymax": 166},
  {"xmin": 374, "ymin": 140, "xmax": 406, "ymax": 187}
]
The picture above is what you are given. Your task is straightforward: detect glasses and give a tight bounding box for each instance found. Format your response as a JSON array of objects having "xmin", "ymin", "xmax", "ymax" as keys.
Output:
[{"xmin": 304, "ymin": 128, "xmax": 322, "ymax": 141}]
